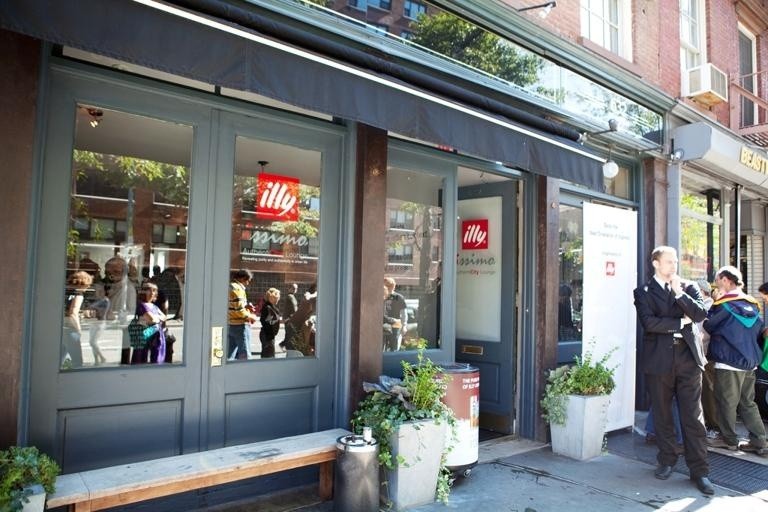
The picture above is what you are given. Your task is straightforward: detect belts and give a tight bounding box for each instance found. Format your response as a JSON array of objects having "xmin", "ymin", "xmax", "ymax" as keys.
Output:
[{"xmin": 671, "ymin": 337, "xmax": 687, "ymax": 345}]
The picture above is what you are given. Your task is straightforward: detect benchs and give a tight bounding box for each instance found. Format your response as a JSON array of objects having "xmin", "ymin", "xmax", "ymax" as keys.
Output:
[{"xmin": 42, "ymin": 422, "xmax": 358, "ymax": 512}]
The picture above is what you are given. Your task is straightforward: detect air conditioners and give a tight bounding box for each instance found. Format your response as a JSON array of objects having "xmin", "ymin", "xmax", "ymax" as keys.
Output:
[{"xmin": 681, "ymin": 61, "xmax": 730, "ymax": 112}]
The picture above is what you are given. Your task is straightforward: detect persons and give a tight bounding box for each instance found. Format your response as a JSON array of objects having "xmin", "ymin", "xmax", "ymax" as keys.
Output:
[
  {"xmin": 383, "ymin": 278, "xmax": 408, "ymax": 352},
  {"xmin": 62, "ymin": 255, "xmax": 184, "ymax": 366},
  {"xmin": 559, "ymin": 284, "xmax": 574, "ymax": 341},
  {"xmin": 227, "ymin": 266, "xmax": 317, "ymax": 361},
  {"xmin": 633, "ymin": 246, "xmax": 768, "ymax": 495}
]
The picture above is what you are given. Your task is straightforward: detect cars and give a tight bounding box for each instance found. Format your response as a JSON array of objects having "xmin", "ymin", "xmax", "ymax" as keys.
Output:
[{"xmin": 303, "ymin": 296, "xmax": 422, "ymax": 353}]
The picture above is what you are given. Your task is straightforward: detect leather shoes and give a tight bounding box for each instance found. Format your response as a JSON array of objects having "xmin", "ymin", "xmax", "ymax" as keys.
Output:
[
  {"xmin": 655, "ymin": 464, "xmax": 673, "ymax": 480},
  {"xmin": 689, "ymin": 474, "xmax": 715, "ymax": 495}
]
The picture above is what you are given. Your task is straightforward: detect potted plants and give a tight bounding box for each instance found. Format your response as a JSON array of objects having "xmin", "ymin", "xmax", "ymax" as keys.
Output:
[
  {"xmin": 1, "ymin": 441, "xmax": 61, "ymax": 512},
  {"xmin": 347, "ymin": 336, "xmax": 462, "ymax": 509},
  {"xmin": 538, "ymin": 333, "xmax": 627, "ymax": 460}
]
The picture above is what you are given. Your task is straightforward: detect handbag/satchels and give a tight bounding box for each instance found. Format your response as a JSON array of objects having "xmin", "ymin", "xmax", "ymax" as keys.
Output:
[{"xmin": 124, "ymin": 318, "xmax": 160, "ymax": 351}]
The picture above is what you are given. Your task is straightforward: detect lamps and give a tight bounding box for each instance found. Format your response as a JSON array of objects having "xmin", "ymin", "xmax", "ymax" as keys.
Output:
[
  {"xmin": 576, "ymin": 121, "xmax": 620, "ymax": 179},
  {"xmin": 518, "ymin": 1, "xmax": 559, "ymax": 22},
  {"xmin": 89, "ymin": 106, "xmax": 105, "ymax": 127},
  {"xmin": 638, "ymin": 137, "xmax": 686, "ymax": 168}
]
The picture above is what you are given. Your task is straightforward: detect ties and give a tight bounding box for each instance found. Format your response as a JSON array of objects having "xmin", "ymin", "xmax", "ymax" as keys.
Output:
[{"xmin": 664, "ymin": 283, "xmax": 670, "ymax": 293}]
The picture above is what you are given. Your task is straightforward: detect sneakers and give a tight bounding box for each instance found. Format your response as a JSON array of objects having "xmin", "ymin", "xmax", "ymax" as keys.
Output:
[
  {"xmin": 703, "ymin": 436, "xmax": 739, "ymax": 452},
  {"xmin": 740, "ymin": 438, "xmax": 768, "ymax": 456}
]
